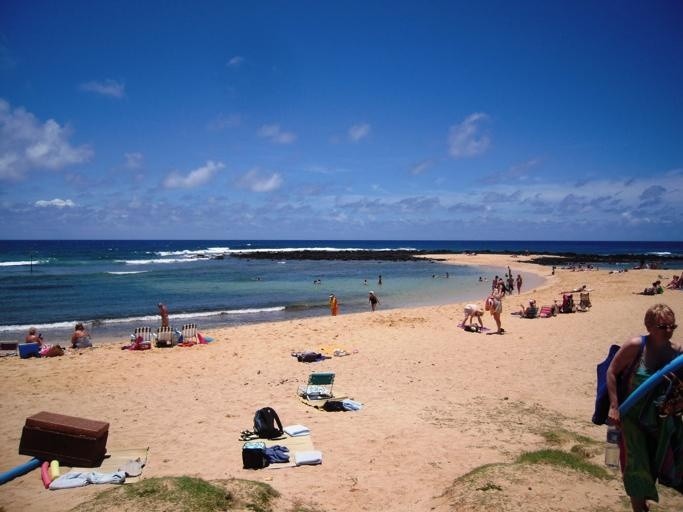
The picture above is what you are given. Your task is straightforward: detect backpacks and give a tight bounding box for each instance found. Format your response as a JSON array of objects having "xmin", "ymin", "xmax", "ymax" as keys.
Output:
[{"xmin": 254, "ymin": 407, "xmax": 284, "ymax": 439}]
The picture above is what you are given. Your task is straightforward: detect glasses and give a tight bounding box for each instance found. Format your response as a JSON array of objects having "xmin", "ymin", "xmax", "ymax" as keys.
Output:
[{"xmin": 657, "ymin": 323, "xmax": 677, "ymax": 329}]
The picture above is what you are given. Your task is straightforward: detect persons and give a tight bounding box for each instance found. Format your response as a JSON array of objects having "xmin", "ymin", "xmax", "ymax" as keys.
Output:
[
  {"xmin": 26, "ymin": 327, "xmax": 41, "ymax": 344},
  {"xmin": 368, "ymin": 291, "xmax": 381, "ymax": 312},
  {"xmin": 329, "ymin": 295, "xmax": 338, "ymax": 316},
  {"xmin": 158, "ymin": 303, "xmax": 169, "ymax": 328},
  {"xmin": 551, "ymin": 263, "xmax": 593, "ymax": 275},
  {"xmin": 462, "ymin": 265, "xmax": 537, "ymax": 334},
  {"xmin": 71, "ymin": 324, "xmax": 92, "ymax": 348},
  {"xmin": 605, "ymin": 301, "xmax": 682, "ymax": 512},
  {"xmin": 643, "ymin": 275, "xmax": 683, "ymax": 296}
]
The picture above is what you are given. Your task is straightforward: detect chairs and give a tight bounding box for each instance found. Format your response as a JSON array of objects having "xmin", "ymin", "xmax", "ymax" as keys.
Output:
[
  {"xmin": 17, "ymin": 341, "xmax": 40, "ymax": 358},
  {"xmin": 155, "ymin": 323, "xmax": 175, "ymax": 348},
  {"xmin": 523, "ymin": 291, "xmax": 593, "ymax": 319},
  {"xmin": 180, "ymin": 323, "xmax": 199, "ymax": 347},
  {"xmin": 129, "ymin": 324, "xmax": 151, "ymax": 349},
  {"xmin": 296, "ymin": 371, "xmax": 336, "ymax": 402}
]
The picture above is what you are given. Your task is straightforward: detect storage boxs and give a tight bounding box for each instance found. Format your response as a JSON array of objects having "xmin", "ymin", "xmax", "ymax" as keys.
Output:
[{"xmin": 16, "ymin": 409, "xmax": 109, "ymax": 468}]
